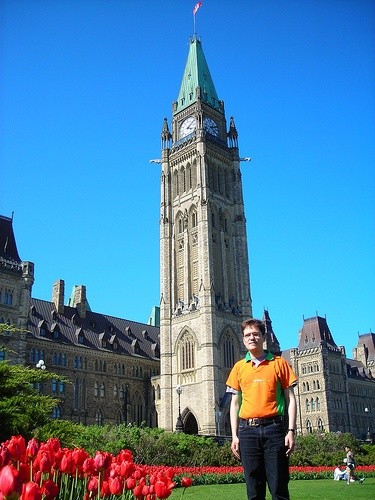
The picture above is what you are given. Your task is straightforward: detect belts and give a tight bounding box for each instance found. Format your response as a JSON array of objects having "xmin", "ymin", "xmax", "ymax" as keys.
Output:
[{"xmin": 237, "ymin": 415, "xmax": 278, "ymax": 427}]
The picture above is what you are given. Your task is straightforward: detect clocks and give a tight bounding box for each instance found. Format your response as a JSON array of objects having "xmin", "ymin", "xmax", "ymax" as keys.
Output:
[
  {"xmin": 179, "ymin": 115, "xmax": 197, "ymax": 139},
  {"xmin": 203, "ymin": 118, "xmax": 219, "ymax": 137}
]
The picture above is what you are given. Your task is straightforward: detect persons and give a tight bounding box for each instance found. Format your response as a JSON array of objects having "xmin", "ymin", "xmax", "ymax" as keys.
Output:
[
  {"xmin": 225, "ymin": 319, "xmax": 299, "ymax": 500},
  {"xmin": 334, "ymin": 446, "xmax": 365, "ymax": 486}
]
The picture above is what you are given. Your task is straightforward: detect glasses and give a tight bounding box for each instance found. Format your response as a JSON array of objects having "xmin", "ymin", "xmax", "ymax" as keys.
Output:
[{"xmin": 241, "ymin": 332, "xmax": 263, "ymax": 340}]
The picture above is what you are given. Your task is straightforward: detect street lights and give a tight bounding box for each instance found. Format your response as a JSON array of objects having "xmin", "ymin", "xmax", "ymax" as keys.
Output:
[
  {"xmin": 365, "ymin": 407, "xmax": 372, "ymax": 439},
  {"xmin": 36, "ymin": 360, "xmax": 46, "ymax": 394},
  {"xmin": 176, "ymin": 385, "xmax": 184, "ymax": 433}
]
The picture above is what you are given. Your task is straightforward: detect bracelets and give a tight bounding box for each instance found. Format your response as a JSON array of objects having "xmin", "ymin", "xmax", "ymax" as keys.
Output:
[{"xmin": 286, "ymin": 429, "xmax": 296, "ymax": 435}]
